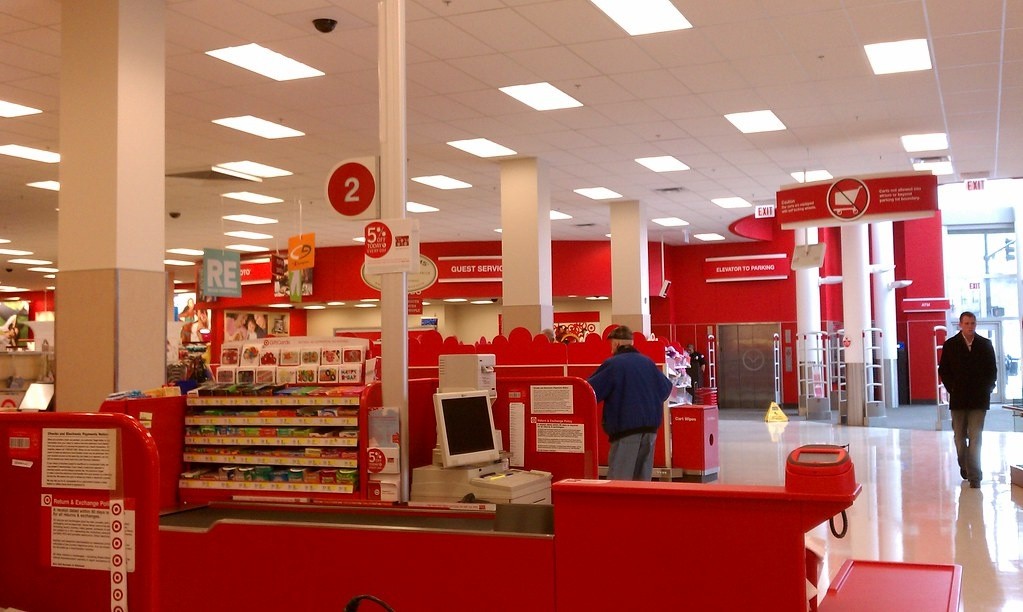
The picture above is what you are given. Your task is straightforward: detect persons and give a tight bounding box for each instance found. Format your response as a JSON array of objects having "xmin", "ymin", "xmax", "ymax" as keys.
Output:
[
  {"xmin": 685, "ymin": 344, "xmax": 706, "ymax": 404},
  {"xmin": 587, "ymin": 325, "xmax": 672, "ymax": 482},
  {"xmin": 0, "ymin": 315, "xmax": 20, "ymax": 352},
  {"xmin": 178, "ymin": 298, "xmax": 208, "ymax": 342},
  {"xmin": 226, "ymin": 314, "xmax": 268, "ymax": 341},
  {"xmin": 938, "ymin": 312, "xmax": 997, "ymax": 488}
]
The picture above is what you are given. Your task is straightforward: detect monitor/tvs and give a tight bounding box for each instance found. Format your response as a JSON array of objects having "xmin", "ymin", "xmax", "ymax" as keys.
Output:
[
  {"xmin": 18, "ymin": 382, "xmax": 56, "ymax": 412},
  {"xmin": 434, "ymin": 390, "xmax": 500, "ymax": 469},
  {"xmin": 791, "ymin": 243, "xmax": 827, "ymax": 270}
]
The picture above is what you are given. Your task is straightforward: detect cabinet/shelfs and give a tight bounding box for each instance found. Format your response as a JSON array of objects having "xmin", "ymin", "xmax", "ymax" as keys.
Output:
[
  {"xmin": 176, "ymin": 382, "xmax": 380, "ymax": 509},
  {"xmin": 0, "ymin": 349, "xmax": 55, "ymax": 413}
]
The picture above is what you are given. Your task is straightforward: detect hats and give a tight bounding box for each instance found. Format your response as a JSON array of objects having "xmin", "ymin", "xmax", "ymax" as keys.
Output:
[{"xmin": 608, "ymin": 326, "xmax": 633, "ymax": 340}]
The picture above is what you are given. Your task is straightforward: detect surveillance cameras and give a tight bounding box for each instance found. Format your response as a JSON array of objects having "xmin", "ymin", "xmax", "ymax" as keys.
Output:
[
  {"xmin": 6, "ymin": 269, "xmax": 13, "ymax": 272},
  {"xmin": 313, "ymin": 18, "xmax": 338, "ymax": 33},
  {"xmin": 169, "ymin": 212, "xmax": 180, "ymax": 218}
]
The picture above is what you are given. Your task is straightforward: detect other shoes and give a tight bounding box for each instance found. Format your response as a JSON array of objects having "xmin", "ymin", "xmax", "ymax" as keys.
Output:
[
  {"xmin": 969, "ymin": 479, "xmax": 980, "ymax": 488},
  {"xmin": 960, "ymin": 469, "xmax": 971, "ymax": 480}
]
